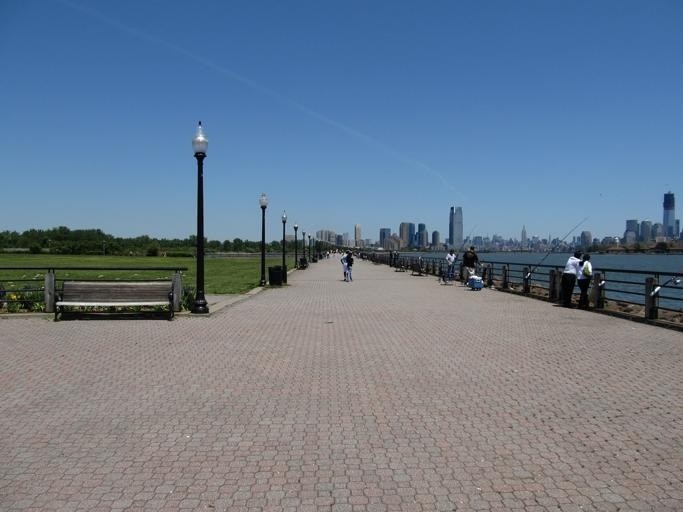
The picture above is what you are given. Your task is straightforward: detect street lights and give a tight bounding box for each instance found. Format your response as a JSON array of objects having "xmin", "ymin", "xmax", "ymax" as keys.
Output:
[
  {"xmin": 258, "ymin": 192, "xmax": 269, "ymax": 288},
  {"xmin": 293, "ymin": 224, "xmax": 298, "ymax": 267},
  {"xmin": 302, "ymin": 231, "xmax": 331, "ymax": 267},
  {"xmin": 280, "ymin": 210, "xmax": 288, "ymax": 282},
  {"xmin": 189, "ymin": 119, "xmax": 210, "ymax": 314}
]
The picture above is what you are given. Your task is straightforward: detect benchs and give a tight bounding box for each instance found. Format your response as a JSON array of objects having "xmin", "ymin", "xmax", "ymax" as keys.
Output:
[{"xmin": 53, "ymin": 280, "xmax": 175, "ymax": 321}]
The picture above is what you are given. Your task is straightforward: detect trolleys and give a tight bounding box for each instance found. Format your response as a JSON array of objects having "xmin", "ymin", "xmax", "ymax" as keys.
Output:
[{"xmin": 466, "ymin": 268, "xmax": 486, "ymax": 292}]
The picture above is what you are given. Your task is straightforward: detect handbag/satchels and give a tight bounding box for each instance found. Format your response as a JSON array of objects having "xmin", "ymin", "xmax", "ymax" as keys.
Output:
[{"xmin": 583, "ymin": 262, "xmax": 591, "ymax": 276}]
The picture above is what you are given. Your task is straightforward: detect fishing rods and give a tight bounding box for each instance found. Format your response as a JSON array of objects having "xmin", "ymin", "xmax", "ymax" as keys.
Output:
[
  {"xmin": 650, "ymin": 273, "xmax": 682, "ymax": 298},
  {"xmin": 448, "ymin": 220, "xmax": 477, "ymax": 279},
  {"xmin": 525, "ymin": 218, "xmax": 587, "ymax": 284}
]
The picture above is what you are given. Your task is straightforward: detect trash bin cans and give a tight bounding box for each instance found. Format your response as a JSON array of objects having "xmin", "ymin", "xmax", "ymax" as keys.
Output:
[
  {"xmin": 268, "ymin": 265, "xmax": 287, "ymax": 286},
  {"xmin": 300, "ymin": 258, "xmax": 307, "ymax": 270},
  {"xmin": 312, "ymin": 253, "xmax": 325, "ymax": 262}
]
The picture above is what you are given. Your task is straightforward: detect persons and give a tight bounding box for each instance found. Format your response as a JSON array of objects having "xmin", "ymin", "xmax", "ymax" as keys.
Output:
[
  {"xmin": 340, "ymin": 250, "xmax": 349, "ymax": 281},
  {"xmin": 462, "ymin": 246, "xmax": 482, "ymax": 287},
  {"xmin": 560, "ymin": 250, "xmax": 582, "ymax": 308},
  {"xmin": 575, "ymin": 254, "xmax": 593, "ymax": 310},
  {"xmin": 345, "ymin": 252, "xmax": 353, "ymax": 282},
  {"xmin": 445, "ymin": 249, "xmax": 457, "ymax": 281}
]
